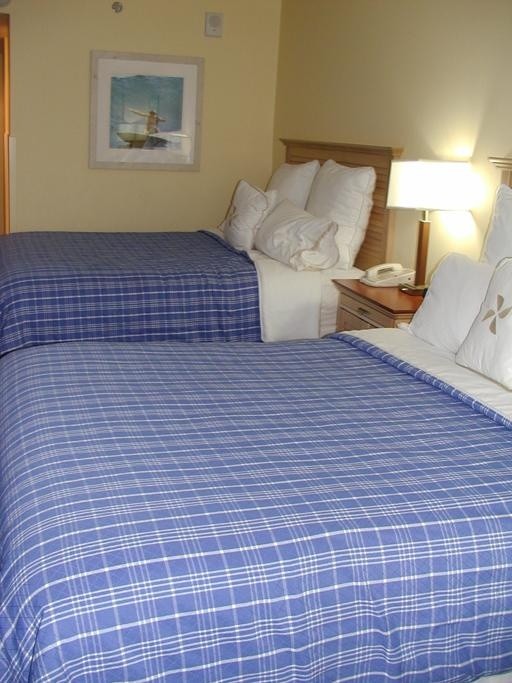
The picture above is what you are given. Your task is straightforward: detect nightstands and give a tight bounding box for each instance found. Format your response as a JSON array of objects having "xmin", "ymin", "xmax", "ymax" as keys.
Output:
[{"xmin": 328, "ymin": 276, "xmax": 424, "ymax": 336}]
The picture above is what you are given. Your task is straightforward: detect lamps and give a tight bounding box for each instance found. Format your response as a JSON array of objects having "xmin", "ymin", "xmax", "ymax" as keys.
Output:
[{"xmin": 385, "ymin": 158, "xmax": 478, "ymax": 294}]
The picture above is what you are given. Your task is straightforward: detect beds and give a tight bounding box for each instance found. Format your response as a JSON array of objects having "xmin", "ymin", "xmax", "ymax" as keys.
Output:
[
  {"xmin": 1, "ymin": 138, "xmax": 404, "ymax": 357},
  {"xmin": 0, "ymin": 157, "xmax": 512, "ymax": 683}
]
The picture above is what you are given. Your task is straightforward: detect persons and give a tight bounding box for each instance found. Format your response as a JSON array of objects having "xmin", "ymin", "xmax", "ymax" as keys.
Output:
[{"xmin": 128, "ymin": 108, "xmax": 166, "ymax": 134}]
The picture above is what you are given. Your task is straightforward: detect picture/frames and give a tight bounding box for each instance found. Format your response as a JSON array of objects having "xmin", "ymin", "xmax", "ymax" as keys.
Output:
[{"xmin": 88, "ymin": 50, "xmax": 203, "ymax": 171}]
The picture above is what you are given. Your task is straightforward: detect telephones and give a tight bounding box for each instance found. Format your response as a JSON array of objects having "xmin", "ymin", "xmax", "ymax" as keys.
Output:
[{"xmin": 360, "ymin": 263, "xmax": 416, "ymax": 288}]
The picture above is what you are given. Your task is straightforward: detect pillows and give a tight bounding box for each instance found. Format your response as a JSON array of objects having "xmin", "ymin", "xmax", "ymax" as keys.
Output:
[
  {"xmin": 223, "ymin": 178, "xmax": 268, "ymax": 253},
  {"xmin": 217, "ymin": 189, "xmax": 280, "ymax": 241},
  {"xmin": 408, "ymin": 250, "xmax": 495, "ymax": 362},
  {"xmin": 479, "ymin": 183, "xmax": 512, "ymax": 268},
  {"xmin": 304, "ymin": 157, "xmax": 376, "ymax": 271},
  {"xmin": 264, "ymin": 158, "xmax": 324, "ymax": 212},
  {"xmin": 253, "ymin": 198, "xmax": 340, "ymax": 272},
  {"xmin": 452, "ymin": 255, "xmax": 512, "ymax": 391}
]
